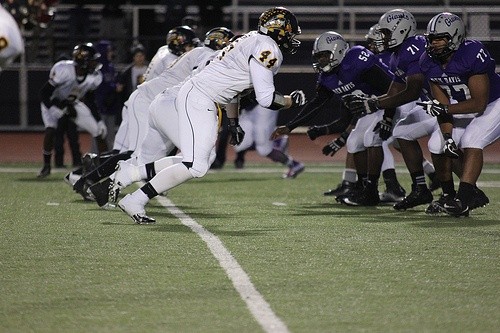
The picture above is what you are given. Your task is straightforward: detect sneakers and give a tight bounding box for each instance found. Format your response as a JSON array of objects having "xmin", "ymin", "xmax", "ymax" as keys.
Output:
[
  {"xmin": 323, "ymin": 180, "xmax": 489, "ymax": 217},
  {"xmin": 118, "ymin": 194, "xmax": 156, "ymax": 225},
  {"xmin": 109, "ymin": 160, "xmax": 139, "ymax": 204}
]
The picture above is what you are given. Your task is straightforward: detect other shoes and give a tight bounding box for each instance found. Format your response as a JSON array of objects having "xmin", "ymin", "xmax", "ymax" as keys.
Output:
[
  {"xmin": 284, "ymin": 162, "xmax": 304, "ymax": 179},
  {"xmin": 38, "ymin": 168, "xmax": 50, "ymax": 177}
]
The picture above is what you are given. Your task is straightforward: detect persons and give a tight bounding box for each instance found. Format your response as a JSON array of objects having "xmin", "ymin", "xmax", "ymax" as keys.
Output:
[
  {"xmin": 234, "ymin": 101, "xmax": 305, "ymax": 178},
  {"xmin": 108, "ymin": 7, "xmax": 306, "ymax": 225},
  {"xmin": 116, "ymin": 44, "xmax": 148, "ymax": 94},
  {"xmin": 268, "ymin": 8, "xmax": 500, "ymax": 216},
  {"xmin": 55, "ymin": 40, "xmax": 117, "ymax": 167},
  {"xmin": 64, "ymin": 25, "xmax": 237, "ymax": 204},
  {"xmin": 38, "ymin": 42, "xmax": 107, "ymax": 177}
]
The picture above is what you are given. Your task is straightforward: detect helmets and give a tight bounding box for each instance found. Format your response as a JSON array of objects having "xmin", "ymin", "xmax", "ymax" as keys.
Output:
[
  {"xmin": 423, "ymin": 13, "xmax": 465, "ymax": 63},
  {"xmin": 377, "ymin": 9, "xmax": 417, "ymax": 50},
  {"xmin": 0, "ymin": 0, "xmax": 55, "ymax": 48},
  {"xmin": 258, "ymin": 7, "xmax": 300, "ymax": 58},
  {"xmin": 311, "ymin": 31, "xmax": 349, "ymax": 74},
  {"xmin": 167, "ymin": 26, "xmax": 197, "ymax": 57},
  {"xmin": 367, "ymin": 24, "xmax": 385, "ymax": 52},
  {"xmin": 73, "ymin": 43, "xmax": 100, "ymax": 74},
  {"xmin": 204, "ymin": 27, "xmax": 235, "ymax": 51},
  {"xmin": 229, "ymin": 34, "xmax": 243, "ymax": 43}
]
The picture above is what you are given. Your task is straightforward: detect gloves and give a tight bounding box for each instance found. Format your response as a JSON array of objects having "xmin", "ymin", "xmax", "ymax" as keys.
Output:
[
  {"xmin": 97, "ymin": 121, "xmax": 108, "ymax": 139},
  {"xmin": 372, "ymin": 116, "xmax": 392, "ymax": 140},
  {"xmin": 341, "ymin": 93, "xmax": 373, "ymax": 112},
  {"xmin": 291, "ymin": 89, "xmax": 307, "ymax": 108},
  {"xmin": 322, "ymin": 136, "xmax": 347, "ymax": 157},
  {"xmin": 228, "ymin": 118, "xmax": 245, "ymax": 146},
  {"xmin": 307, "ymin": 125, "xmax": 329, "ymax": 140},
  {"xmin": 50, "ymin": 107, "xmax": 62, "ymax": 119},
  {"xmin": 415, "ymin": 99, "xmax": 448, "ymax": 117},
  {"xmin": 443, "ymin": 139, "xmax": 464, "ymax": 159},
  {"xmin": 348, "ymin": 96, "xmax": 382, "ymax": 117}
]
